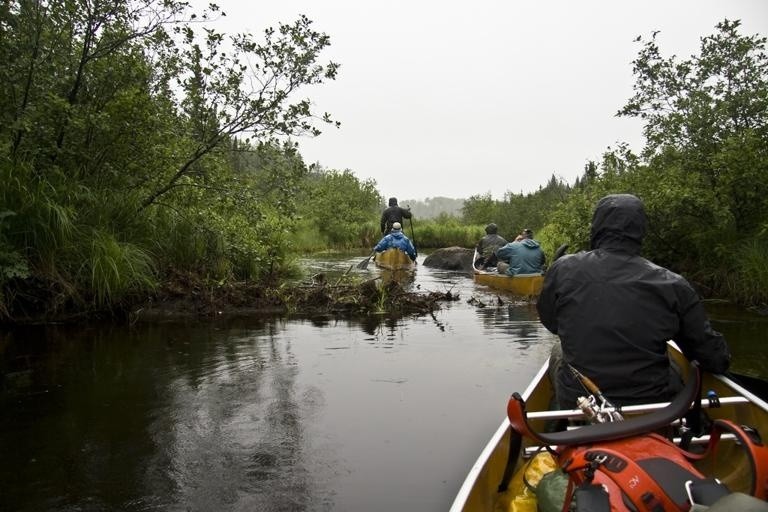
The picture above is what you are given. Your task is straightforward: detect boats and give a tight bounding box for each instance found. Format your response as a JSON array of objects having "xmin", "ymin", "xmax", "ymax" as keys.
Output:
[
  {"xmin": 449, "ymin": 337, "xmax": 767, "ymax": 511},
  {"xmin": 471, "ymin": 247, "xmax": 545, "ymax": 297},
  {"xmin": 373, "ymin": 250, "xmax": 413, "ymax": 270}
]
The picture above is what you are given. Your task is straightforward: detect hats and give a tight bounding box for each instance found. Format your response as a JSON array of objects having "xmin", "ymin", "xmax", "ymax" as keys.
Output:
[{"xmin": 390, "ymin": 221, "xmax": 404, "ymax": 233}]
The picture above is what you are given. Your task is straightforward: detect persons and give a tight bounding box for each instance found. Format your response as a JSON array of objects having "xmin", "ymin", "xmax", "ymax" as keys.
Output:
[
  {"xmin": 474, "ymin": 223, "xmax": 509, "ymax": 268},
  {"xmin": 374, "ymin": 223, "xmax": 416, "ymax": 262},
  {"xmin": 536, "ymin": 192, "xmax": 733, "ymax": 430},
  {"xmin": 496, "ymin": 228, "xmax": 545, "ymax": 276},
  {"xmin": 381, "ymin": 198, "xmax": 412, "ymax": 237}
]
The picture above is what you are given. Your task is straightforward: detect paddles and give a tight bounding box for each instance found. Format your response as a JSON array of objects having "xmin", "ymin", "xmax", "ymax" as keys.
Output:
[{"xmin": 357, "ymin": 250, "xmax": 375, "ymax": 269}]
